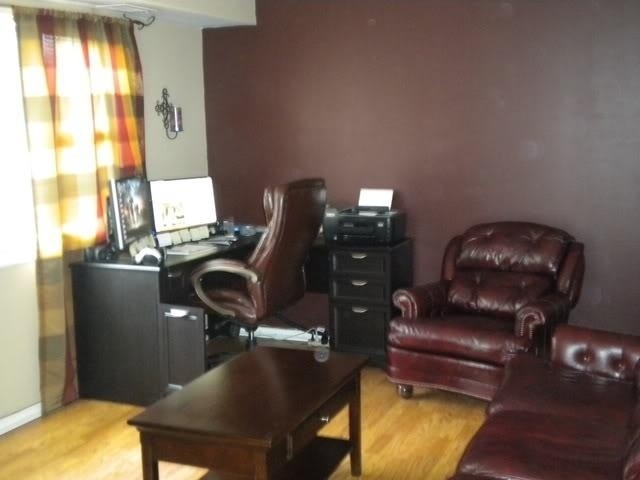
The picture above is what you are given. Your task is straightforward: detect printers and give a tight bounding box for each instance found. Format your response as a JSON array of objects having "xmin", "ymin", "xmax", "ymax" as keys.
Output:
[{"xmin": 323, "ymin": 206, "xmax": 406, "ymax": 246}]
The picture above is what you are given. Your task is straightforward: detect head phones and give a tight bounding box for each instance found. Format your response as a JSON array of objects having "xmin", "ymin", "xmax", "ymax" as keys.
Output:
[
  {"xmin": 84, "ymin": 240, "xmax": 116, "ymax": 263},
  {"xmin": 132, "ymin": 248, "xmax": 166, "ymax": 267}
]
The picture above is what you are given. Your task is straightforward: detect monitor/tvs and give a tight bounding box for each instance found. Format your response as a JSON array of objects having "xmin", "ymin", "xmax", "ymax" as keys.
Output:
[
  {"xmin": 106, "ymin": 174, "xmax": 154, "ymax": 251},
  {"xmin": 149, "ymin": 176, "xmax": 219, "ymax": 247}
]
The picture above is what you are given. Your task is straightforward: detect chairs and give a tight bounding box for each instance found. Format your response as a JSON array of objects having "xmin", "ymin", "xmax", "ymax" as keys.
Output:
[
  {"xmin": 380, "ymin": 216, "xmax": 588, "ymax": 413},
  {"xmin": 194, "ymin": 174, "xmax": 328, "ymax": 367}
]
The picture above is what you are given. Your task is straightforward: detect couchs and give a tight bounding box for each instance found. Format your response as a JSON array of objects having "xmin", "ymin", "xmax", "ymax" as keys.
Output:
[{"xmin": 452, "ymin": 321, "xmax": 640, "ymax": 480}]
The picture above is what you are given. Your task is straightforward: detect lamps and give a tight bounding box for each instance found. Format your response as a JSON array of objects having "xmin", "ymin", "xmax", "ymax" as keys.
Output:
[{"xmin": 153, "ymin": 86, "xmax": 183, "ymax": 141}]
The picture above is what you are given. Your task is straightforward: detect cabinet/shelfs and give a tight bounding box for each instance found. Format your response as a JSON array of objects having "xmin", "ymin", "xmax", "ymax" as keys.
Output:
[{"xmin": 326, "ymin": 239, "xmax": 416, "ymax": 369}]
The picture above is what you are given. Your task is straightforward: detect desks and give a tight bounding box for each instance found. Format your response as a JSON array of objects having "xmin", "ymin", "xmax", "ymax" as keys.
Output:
[{"xmin": 66, "ymin": 226, "xmax": 271, "ymax": 409}]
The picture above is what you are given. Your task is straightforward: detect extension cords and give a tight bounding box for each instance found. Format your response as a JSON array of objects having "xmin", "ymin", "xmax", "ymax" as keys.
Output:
[{"xmin": 308, "ymin": 339, "xmax": 329, "ymax": 347}]
